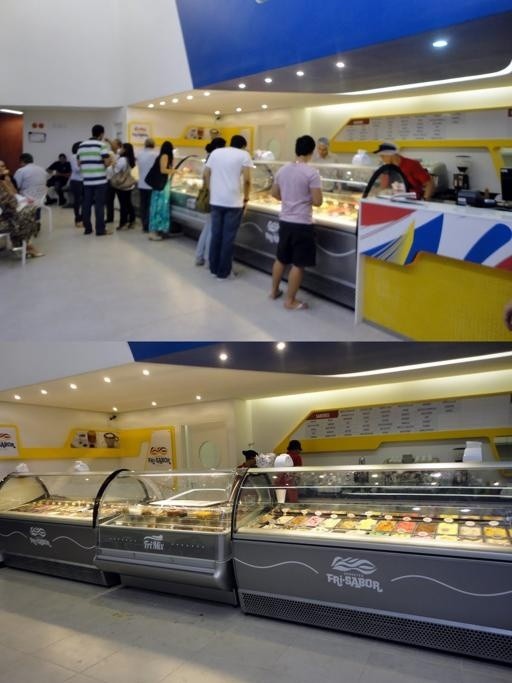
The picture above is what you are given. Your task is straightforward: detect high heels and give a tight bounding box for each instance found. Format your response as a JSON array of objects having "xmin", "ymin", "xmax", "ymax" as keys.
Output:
[{"xmin": 26, "ymin": 248, "xmax": 45, "ymax": 257}]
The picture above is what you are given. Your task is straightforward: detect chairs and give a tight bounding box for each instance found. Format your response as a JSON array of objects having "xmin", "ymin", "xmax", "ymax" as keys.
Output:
[{"xmin": 1, "ymin": 169, "xmax": 77, "ymax": 265}]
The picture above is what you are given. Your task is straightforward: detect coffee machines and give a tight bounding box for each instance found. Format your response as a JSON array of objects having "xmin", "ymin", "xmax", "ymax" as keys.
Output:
[
  {"xmin": 450, "ymin": 447, "xmax": 467, "ymax": 488},
  {"xmin": 451, "ymin": 156, "xmax": 469, "ymax": 193}
]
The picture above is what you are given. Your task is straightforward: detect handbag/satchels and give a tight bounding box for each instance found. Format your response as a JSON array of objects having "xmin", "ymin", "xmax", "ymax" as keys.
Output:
[
  {"xmin": 195, "ymin": 187, "xmax": 210, "ymax": 213},
  {"xmin": 144, "ymin": 166, "xmax": 168, "ymax": 190}
]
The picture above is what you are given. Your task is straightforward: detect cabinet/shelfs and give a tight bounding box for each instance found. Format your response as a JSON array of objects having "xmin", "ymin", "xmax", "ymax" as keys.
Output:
[
  {"xmin": 225, "ymin": 155, "xmax": 411, "ymax": 312},
  {"xmin": 228, "ymin": 459, "xmax": 511, "ymax": 668},
  {"xmin": 90, "ymin": 466, "xmax": 251, "ymax": 610},
  {"xmin": 129, "ymin": 148, "xmax": 198, "ymax": 235},
  {"xmin": 1, "ymin": 469, "xmax": 151, "ymax": 590},
  {"xmin": 166, "ymin": 153, "xmax": 275, "ymax": 249}
]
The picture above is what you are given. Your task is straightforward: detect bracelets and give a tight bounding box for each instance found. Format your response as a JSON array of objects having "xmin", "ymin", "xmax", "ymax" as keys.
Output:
[
  {"xmin": 243, "ymin": 198, "xmax": 250, "ymax": 203},
  {"xmin": 422, "ymin": 196, "xmax": 427, "ymax": 200}
]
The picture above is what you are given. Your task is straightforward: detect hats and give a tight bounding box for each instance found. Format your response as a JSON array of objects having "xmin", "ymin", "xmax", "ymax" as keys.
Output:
[
  {"xmin": 371, "ymin": 142, "xmax": 396, "ymax": 153},
  {"xmin": 0, "ymin": 160, "xmax": 10, "ymax": 174}
]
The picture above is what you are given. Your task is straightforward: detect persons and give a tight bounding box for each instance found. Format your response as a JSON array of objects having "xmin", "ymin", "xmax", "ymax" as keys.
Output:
[
  {"xmin": 193, "ymin": 137, "xmax": 226, "ymax": 270},
  {"xmin": 371, "ymin": 142, "xmax": 436, "ymax": 200},
  {"xmin": 136, "ymin": 138, "xmax": 161, "ymax": 232},
  {"xmin": 145, "ymin": 140, "xmax": 182, "ymax": 240},
  {"xmin": 236, "ymin": 439, "xmax": 303, "ymax": 503},
  {"xmin": 312, "ymin": 136, "xmax": 343, "ymax": 193},
  {"xmin": 0, "ymin": 124, "xmax": 136, "ymax": 257},
  {"xmin": 203, "ymin": 133, "xmax": 255, "ymax": 282},
  {"xmin": 267, "ymin": 135, "xmax": 325, "ymax": 312}
]
